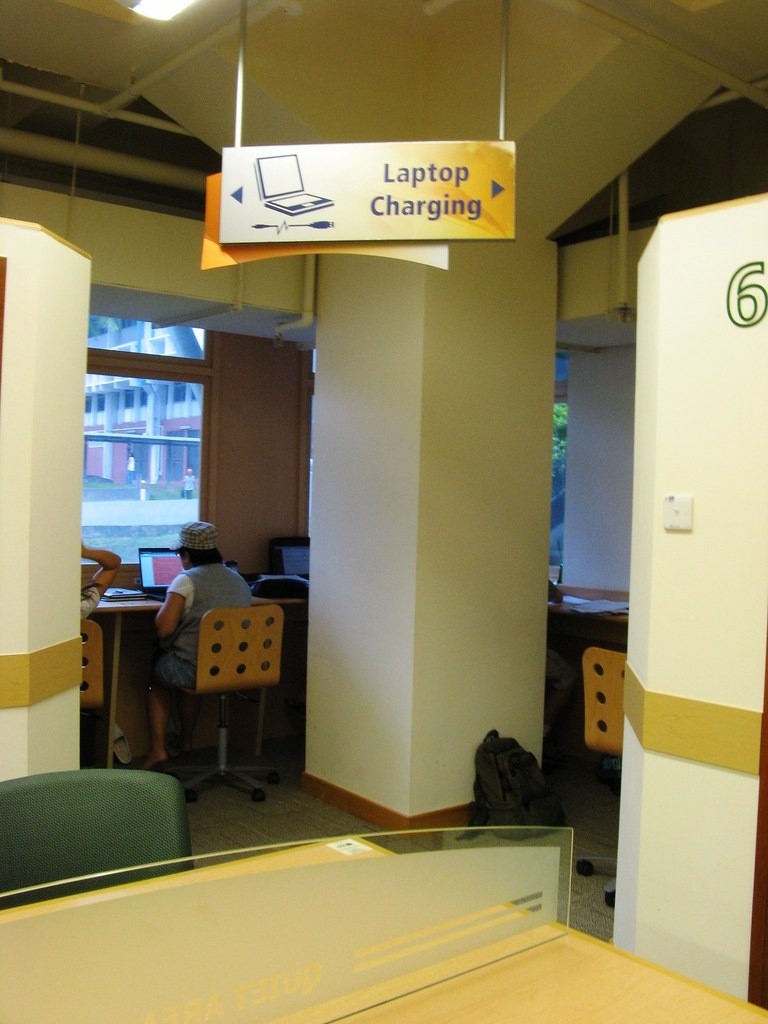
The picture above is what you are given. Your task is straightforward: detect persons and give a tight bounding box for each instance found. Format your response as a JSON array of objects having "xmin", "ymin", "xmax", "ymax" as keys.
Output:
[
  {"xmin": 183, "ymin": 469, "xmax": 198, "ymax": 499},
  {"xmin": 139, "ymin": 523, "xmax": 253, "ymax": 771},
  {"xmin": 126, "ymin": 453, "xmax": 135, "ymax": 484},
  {"xmin": 543, "ymin": 580, "xmax": 577, "ymax": 743},
  {"xmin": 80, "ymin": 545, "xmax": 122, "ymax": 621}
]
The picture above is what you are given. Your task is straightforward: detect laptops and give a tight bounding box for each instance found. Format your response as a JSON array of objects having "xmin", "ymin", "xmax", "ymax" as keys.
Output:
[
  {"xmin": 272, "ymin": 542, "xmax": 309, "ymax": 582},
  {"xmin": 138, "ymin": 547, "xmax": 186, "ymax": 602}
]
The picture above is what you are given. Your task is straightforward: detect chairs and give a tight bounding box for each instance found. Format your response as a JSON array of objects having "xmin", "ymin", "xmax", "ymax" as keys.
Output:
[
  {"xmin": 80, "ymin": 619, "xmax": 104, "ymax": 767},
  {"xmin": 163, "ymin": 603, "xmax": 285, "ymax": 804},
  {"xmin": 1, "ymin": 768, "xmax": 192, "ymax": 913},
  {"xmin": 573, "ymin": 647, "xmax": 627, "ymax": 906}
]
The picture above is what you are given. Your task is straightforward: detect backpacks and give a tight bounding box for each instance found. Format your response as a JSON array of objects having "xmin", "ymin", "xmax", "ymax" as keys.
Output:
[{"xmin": 462, "ymin": 728, "xmax": 564, "ymax": 841}]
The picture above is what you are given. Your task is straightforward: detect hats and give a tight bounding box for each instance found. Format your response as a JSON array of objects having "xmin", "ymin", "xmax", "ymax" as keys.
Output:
[{"xmin": 169, "ymin": 521, "xmax": 217, "ymax": 551}]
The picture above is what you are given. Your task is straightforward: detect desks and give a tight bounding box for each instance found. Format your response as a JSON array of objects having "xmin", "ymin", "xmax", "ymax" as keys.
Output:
[
  {"xmin": 0, "ymin": 829, "xmax": 768, "ymax": 1024},
  {"xmin": 546, "ymin": 598, "xmax": 630, "ymax": 626},
  {"xmin": 79, "ymin": 597, "xmax": 307, "ymax": 769}
]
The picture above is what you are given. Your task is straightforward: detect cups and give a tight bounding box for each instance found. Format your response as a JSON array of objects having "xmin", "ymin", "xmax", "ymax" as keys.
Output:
[{"xmin": 222, "ymin": 559, "xmax": 239, "ymax": 573}]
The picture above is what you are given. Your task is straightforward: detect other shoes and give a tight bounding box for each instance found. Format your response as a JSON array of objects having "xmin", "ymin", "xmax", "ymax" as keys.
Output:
[{"xmin": 112, "ymin": 724, "xmax": 133, "ymax": 765}]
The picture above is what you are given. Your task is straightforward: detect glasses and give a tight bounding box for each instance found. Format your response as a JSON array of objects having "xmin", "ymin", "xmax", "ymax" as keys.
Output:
[{"xmin": 175, "ymin": 552, "xmax": 185, "ymax": 558}]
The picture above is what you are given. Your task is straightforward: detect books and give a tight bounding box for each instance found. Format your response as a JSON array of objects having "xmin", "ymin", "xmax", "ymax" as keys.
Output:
[{"xmin": 100, "ymin": 588, "xmax": 146, "ymax": 602}]
